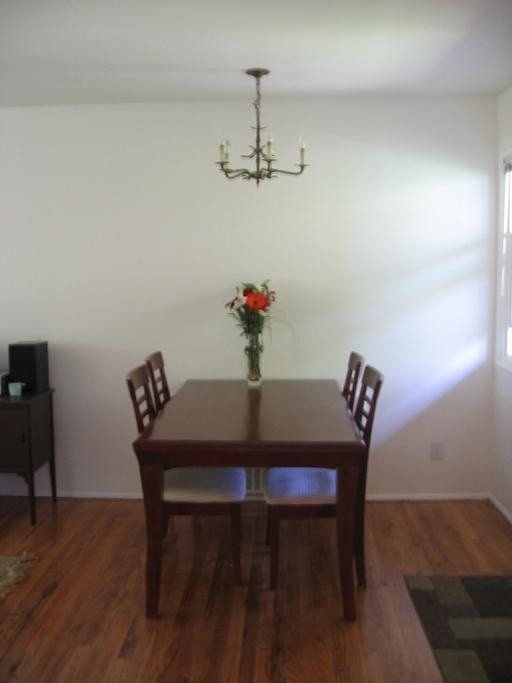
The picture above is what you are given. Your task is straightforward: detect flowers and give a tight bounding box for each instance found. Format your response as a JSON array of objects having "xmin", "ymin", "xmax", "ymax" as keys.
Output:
[{"xmin": 226, "ymin": 279, "xmax": 279, "ymax": 341}]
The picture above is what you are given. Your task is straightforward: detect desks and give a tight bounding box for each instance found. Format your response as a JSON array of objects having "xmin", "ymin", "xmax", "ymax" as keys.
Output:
[
  {"xmin": 1, "ymin": 388, "xmax": 59, "ymax": 527},
  {"xmin": 130, "ymin": 376, "xmax": 368, "ymax": 625}
]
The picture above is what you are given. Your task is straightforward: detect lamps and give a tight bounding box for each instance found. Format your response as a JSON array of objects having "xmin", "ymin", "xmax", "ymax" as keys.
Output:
[{"xmin": 213, "ymin": 67, "xmax": 311, "ymax": 190}]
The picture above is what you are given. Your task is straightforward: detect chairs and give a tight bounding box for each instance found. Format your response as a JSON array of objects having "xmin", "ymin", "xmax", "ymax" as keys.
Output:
[
  {"xmin": 124, "ymin": 363, "xmax": 248, "ymax": 589},
  {"xmin": 258, "ymin": 365, "xmax": 385, "ymax": 593},
  {"xmin": 340, "ymin": 350, "xmax": 364, "ymax": 412},
  {"xmin": 146, "ymin": 350, "xmax": 173, "ymax": 412}
]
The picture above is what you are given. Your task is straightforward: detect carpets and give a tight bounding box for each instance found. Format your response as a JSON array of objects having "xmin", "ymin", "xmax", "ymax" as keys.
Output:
[{"xmin": 400, "ymin": 571, "xmax": 511, "ymax": 682}]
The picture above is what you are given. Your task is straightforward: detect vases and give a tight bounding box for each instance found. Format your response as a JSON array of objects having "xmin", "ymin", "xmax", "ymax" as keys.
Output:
[{"xmin": 244, "ymin": 335, "xmax": 265, "ymax": 388}]
